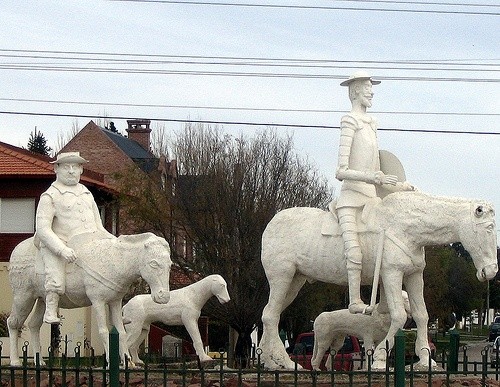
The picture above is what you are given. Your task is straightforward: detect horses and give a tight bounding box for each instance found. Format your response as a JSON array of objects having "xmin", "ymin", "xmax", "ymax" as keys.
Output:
[
  {"xmin": 310, "ymin": 289, "xmax": 414, "ymax": 372},
  {"xmin": 255, "ymin": 188, "xmax": 500, "ymax": 380},
  {"xmin": 6, "ymin": 229, "xmax": 175, "ymax": 368}
]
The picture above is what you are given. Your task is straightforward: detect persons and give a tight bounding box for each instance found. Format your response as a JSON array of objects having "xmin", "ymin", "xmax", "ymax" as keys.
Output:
[
  {"xmin": 333, "ymin": 76, "xmax": 416, "ymax": 314},
  {"xmin": 31, "ymin": 150, "xmax": 108, "ymax": 323}
]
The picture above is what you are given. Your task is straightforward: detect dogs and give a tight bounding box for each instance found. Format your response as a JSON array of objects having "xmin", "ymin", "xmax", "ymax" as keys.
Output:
[{"xmin": 119, "ymin": 273, "xmax": 233, "ymax": 367}]
